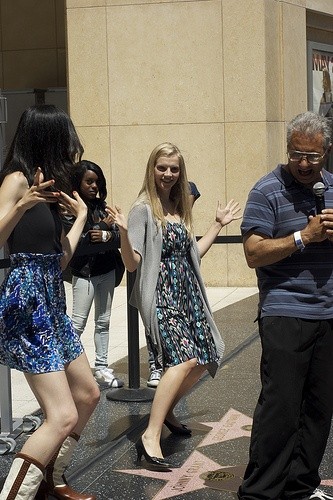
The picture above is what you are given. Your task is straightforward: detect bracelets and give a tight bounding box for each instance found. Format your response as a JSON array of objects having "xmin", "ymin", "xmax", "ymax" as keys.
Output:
[
  {"xmin": 293, "ymin": 231, "xmax": 306, "ymax": 251},
  {"xmin": 216, "ymin": 217, "xmax": 224, "ymax": 228},
  {"xmin": 102, "ymin": 230, "xmax": 111, "ymax": 242}
]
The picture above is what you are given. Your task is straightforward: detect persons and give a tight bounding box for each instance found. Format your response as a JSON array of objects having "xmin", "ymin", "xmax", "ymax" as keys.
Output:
[
  {"xmin": 236, "ymin": 111, "xmax": 333, "ymax": 500},
  {"xmin": 0, "ymin": 103, "xmax": 102, "ymax": 500},
  {"xmin": 105, "ymin": 141, "xmax": 243, "ymax": 469},
  {"xmin": 62, "ymin": 160, "xmax": 124, "ymax": 390}
]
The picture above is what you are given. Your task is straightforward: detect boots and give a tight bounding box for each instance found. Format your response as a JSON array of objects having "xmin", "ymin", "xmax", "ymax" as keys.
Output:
[
  {"xmin": 37, "ymin": 432, "xmax": 96, "ymax": 500},
  {"xmin": 0, "ymin": 452, "xmax": 48, "ymax": 500}
]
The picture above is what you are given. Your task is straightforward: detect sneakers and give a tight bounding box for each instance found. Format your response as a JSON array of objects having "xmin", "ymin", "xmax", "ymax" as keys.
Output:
[
  {"xmin": 94, "ymin": 366, "xmax": 124, "ymax": 388},
  {"xmin": 146, "ymin": 368, "xmax": 162, "ymax": 387}
]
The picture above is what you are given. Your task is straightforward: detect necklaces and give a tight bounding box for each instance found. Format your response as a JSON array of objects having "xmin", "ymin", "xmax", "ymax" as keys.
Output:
[{"xmin": 162, "ymin": 206, "xmax": 180, "ymax": 222}]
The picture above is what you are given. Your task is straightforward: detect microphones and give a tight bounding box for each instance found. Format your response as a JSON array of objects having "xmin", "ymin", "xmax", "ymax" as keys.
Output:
[{"xmin": 312, "ymin": 181, "xmax": 325, "ymax": 214}]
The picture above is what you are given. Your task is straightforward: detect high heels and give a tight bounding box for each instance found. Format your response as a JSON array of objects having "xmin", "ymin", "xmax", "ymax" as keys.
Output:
[
  {"xmin": 135, "ymin": 437, "xmax": 174, "ymax": 468},
  {"xmin": 163, "ymin": 419, "xmax": 192, "ymax": 438}
]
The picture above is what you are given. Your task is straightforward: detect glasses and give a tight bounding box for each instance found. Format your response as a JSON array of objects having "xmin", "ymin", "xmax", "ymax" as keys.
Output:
[{"xmin": 287, "ymin": 148, "xmax": 328, "ymax": 164}]
